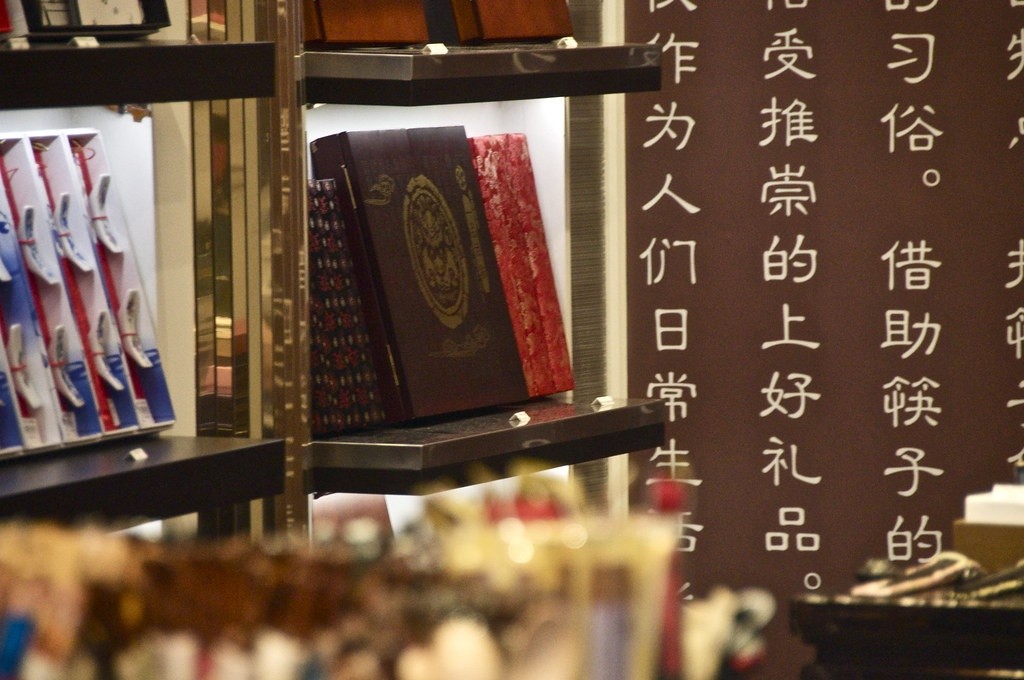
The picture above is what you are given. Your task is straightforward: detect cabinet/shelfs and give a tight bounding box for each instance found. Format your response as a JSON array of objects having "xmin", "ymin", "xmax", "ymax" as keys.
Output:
[
  {"xmin": 307, "ymin": 40, "xmax": 662, "ymax": 491},
  {"xmin": 0, "ymin": 41, "xmax": 277, "ymax": 533}
]
